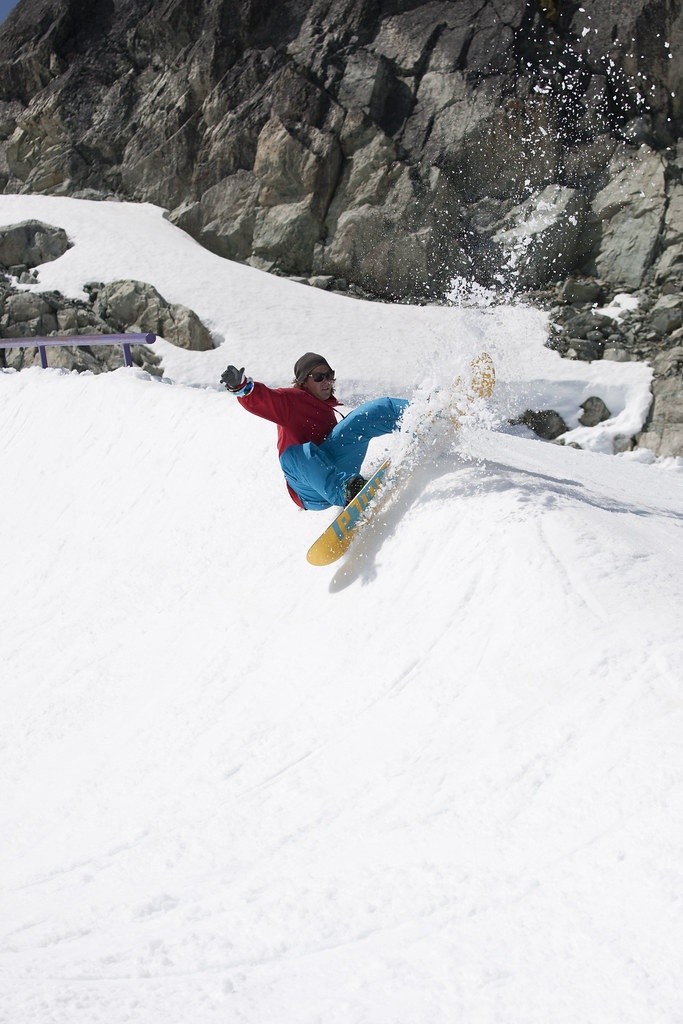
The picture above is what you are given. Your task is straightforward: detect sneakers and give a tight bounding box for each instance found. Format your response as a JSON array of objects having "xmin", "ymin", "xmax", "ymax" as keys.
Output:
[{"xmin": 346, "ymin": 475, "xmax": 364, "ymax": 507}]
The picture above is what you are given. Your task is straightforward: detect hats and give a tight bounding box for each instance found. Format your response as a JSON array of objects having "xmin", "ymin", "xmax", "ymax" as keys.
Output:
[{"xmin": 294, "ymin": 352, "xmax": 328, "ymax": 383}]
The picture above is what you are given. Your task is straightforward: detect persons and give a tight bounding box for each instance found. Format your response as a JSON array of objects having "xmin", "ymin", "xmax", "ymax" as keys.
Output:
[{"xmin": 221, "ymin": 352, "xmax": 415, "ymax": 511}]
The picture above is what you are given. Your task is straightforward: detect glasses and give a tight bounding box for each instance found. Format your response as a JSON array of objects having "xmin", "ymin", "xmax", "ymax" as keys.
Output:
[{"xmin": 308, "ymin": 371, "xmax": 334, "ymax": 382}]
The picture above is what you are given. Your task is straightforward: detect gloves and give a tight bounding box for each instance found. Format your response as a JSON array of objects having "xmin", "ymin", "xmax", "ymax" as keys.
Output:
[{"xmin": 220, "ymin": 365, "xmax": 247, "ymax": 392}]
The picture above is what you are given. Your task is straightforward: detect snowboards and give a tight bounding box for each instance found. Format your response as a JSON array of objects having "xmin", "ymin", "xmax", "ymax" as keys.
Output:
[{"xmin": 306, "ymin": 355, "xmax": 497, "ymax": 568}]
